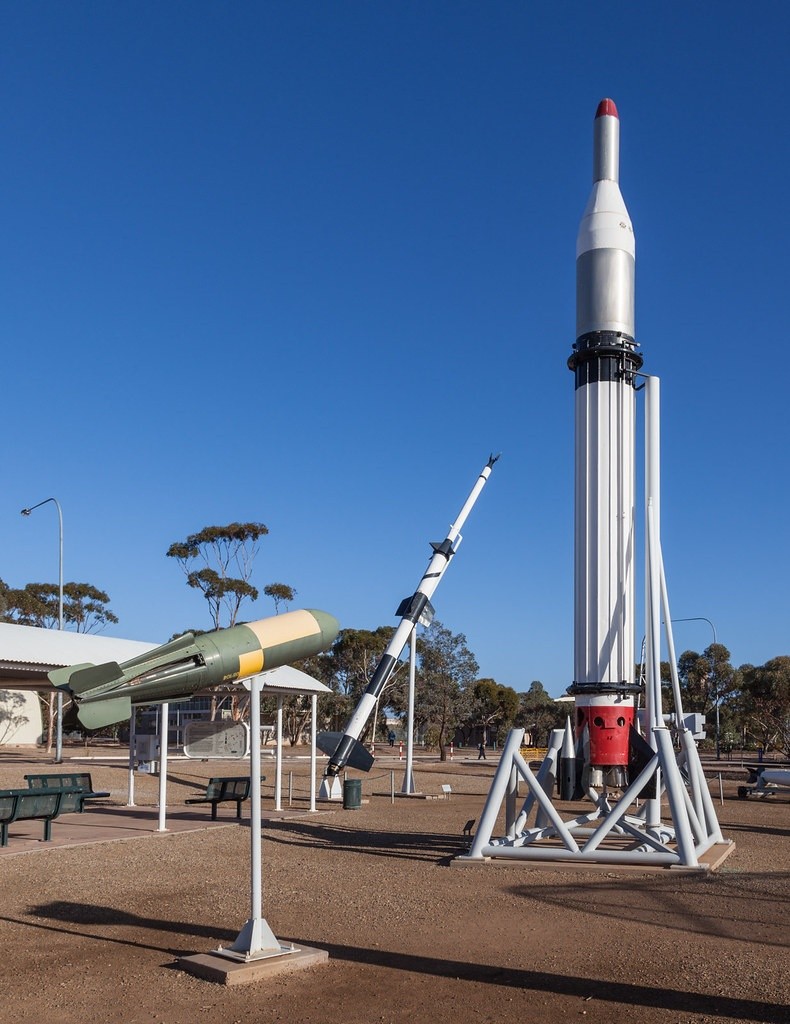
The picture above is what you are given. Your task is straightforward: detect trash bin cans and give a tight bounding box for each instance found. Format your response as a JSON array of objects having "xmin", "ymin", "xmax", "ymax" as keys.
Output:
[{"xmin": 342, "ymin": 778, "xmax": 362, "ymax": 810}]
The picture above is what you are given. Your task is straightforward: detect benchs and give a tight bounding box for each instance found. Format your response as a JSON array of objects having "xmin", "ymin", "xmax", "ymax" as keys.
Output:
[
  {"xmin": 0, "ymin": 785, "xmax": 87, "ymax": 848},
  {"xmin": 24, "ymin": 772, "xmax": 111, "ymax": 814},
  {"xmin": 185, "ymin": 776, "xmax": 266, "ymax": 821}
]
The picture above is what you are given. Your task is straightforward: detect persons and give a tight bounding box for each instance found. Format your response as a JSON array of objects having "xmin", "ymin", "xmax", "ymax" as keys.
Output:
[
  {"xmin": 386, "ymin": 729, "xmax": 391, "ymax": 743},
  {"xmin": 477, "ymin": 741, "xmax": 487, "ymax": 760},
  {"xmin": 389, "ymin": 731, "xmax": 395, "ymax": 748}
]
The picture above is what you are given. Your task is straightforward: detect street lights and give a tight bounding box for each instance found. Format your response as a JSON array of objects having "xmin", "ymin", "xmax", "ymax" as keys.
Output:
[{"xmin": 19, "ymin": 495, "xmax": 66, "ymax": 762}]
